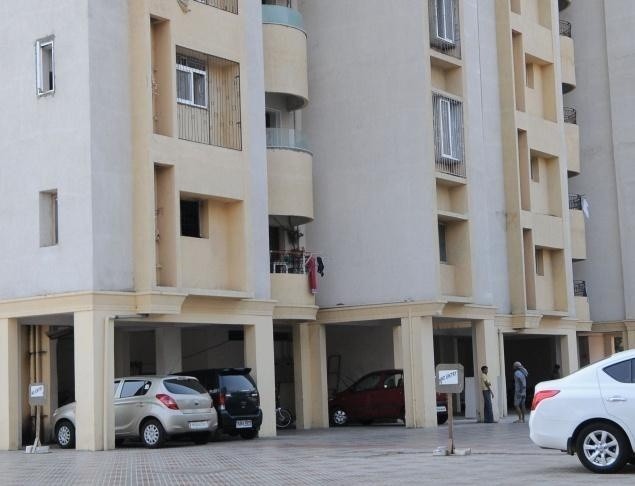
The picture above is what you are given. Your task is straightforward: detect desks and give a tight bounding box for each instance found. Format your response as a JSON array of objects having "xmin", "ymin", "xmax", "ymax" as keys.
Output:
[{"xmin": 272, "ymin": 262, "xmax": 289, "ymax": 273}]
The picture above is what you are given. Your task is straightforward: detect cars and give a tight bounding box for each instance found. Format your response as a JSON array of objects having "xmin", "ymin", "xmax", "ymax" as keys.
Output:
[
  {"xmin": 527, "ymin": 348, "xmax": 634, "ymax": 474},
  {"xmin": 329, "ymin": 369, "xmax": 449, "ymax": 426},
  {"xmin": 171, "ymin": 367, "xmax": 264, "ymax": 444},
  {"xmin": 51, "ymin": 373, "xmax": 220, "ymax": 450}
]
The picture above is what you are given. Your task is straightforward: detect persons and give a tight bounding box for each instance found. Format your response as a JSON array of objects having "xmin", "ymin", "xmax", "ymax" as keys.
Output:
[
  {"xmin": 480, "ymin": 366, "xmax": 498, "ymax": 423},
  {"xmin": 513, "ymin": 362, "xmax": 527, "ymax": 422}
]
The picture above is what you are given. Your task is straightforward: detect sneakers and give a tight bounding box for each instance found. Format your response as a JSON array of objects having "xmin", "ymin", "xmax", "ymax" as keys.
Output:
[{"xmin": 513, "ymin": 420, "xmax": 525, "ymax": 423}]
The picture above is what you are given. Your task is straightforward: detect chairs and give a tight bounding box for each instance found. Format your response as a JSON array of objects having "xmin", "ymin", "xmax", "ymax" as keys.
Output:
[{"xmin": 388, "ymin": 379, "xmax": 402, "ymax": 388}]
